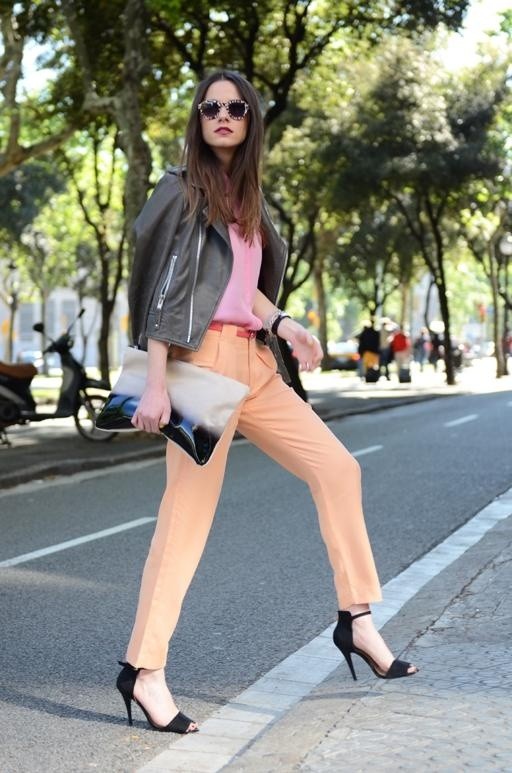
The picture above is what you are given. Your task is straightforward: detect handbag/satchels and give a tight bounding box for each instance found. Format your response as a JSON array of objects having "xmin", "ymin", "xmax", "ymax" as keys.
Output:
[{"xmin": 94, "ymin": 345, "xmax": 250, "ymax": 469}]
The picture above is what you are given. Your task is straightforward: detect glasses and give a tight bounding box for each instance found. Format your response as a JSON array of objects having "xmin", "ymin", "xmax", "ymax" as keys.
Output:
[{"xmin": 198, "ymin": 99, "xmax": 251, "ymax": 121}]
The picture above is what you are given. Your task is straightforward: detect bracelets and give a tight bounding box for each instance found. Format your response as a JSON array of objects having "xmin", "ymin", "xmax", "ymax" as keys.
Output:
[{"xmin": 269, "ymin": 311, "xmax": 291, "ymax": 334}]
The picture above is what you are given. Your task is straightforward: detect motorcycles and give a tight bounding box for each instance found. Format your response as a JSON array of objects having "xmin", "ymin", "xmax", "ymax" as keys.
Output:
[{"xmin": 0, "ymin": 307, "xmax": 122, "ymax": 449}]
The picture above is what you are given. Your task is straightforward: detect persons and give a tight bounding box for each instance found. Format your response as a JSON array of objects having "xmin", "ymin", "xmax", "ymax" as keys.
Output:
[
  {"xmin": 354, "ymin": 316, "xmax": 461, "ymax": 384},
  {"xmin": 114, "ymin": 70, "xmax": 421, "ymax": 734}
]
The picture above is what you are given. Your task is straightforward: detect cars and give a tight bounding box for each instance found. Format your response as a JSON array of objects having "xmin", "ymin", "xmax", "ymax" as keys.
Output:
[{"xmin": 324, "ymin": 339, "xmax": 358, "ymax": 373}]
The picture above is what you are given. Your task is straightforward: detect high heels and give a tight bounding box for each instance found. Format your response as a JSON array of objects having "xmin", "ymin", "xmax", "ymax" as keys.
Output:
[
  {"xmin": 332, "ymin": 609, "xmax": 420, "ymax": 682},
  {"xmin": 115, "ymin": 659, "xmax": 200, "ymax": 735}
]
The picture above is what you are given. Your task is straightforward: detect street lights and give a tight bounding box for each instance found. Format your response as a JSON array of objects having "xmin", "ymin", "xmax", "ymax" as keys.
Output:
[{"xmin": 499, "ymin": 234, "xmax": 512, "ymax": 374}]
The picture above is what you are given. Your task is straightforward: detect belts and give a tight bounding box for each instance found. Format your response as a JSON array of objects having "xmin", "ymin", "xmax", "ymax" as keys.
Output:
[{"xmin": 208, "ymin": 321, "xmax": 258, "ymax": 339}]
